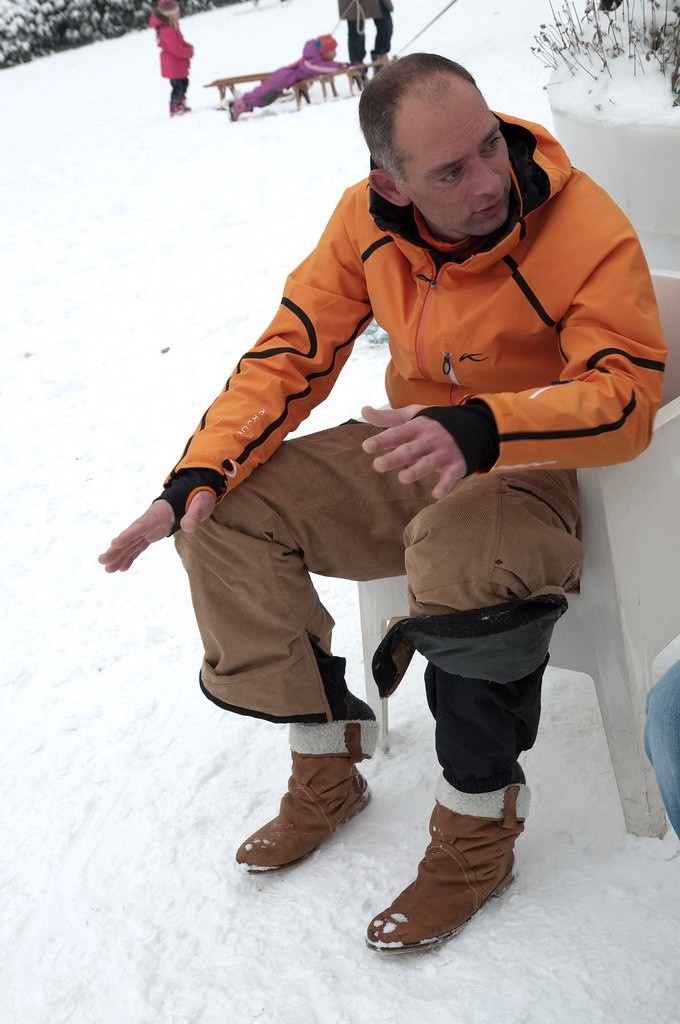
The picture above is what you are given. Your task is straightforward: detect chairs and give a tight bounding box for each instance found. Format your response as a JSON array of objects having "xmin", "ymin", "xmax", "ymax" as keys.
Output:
[{"xmin": 355, "ymin": 264, "xmax": 680, "ymax": 844}]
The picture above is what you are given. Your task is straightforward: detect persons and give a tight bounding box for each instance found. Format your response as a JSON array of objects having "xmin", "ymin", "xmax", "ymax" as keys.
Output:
[
  {"xmin": 338, "ymin": 0, "xmax": 394, "ymax": 80},
  {"xmin": 98, "ymin": 54, "xmax": 669, "ymax": 955},
  {"xmin": 148, "ymin": 0, "xmax": 195, "ymax": 114},
  {"xmin": 227, "ymin": 34, "xmax": 367, "ymax": 122}
]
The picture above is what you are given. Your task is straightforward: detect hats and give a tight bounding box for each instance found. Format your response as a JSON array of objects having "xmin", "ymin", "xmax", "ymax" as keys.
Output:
[
  {"xmin": 157, "ymin": 0, "xmax": 180, "ymax": 17},
  {"xmin": 314, "ymin": 35, "xmax": 337, "ymax": 54}
]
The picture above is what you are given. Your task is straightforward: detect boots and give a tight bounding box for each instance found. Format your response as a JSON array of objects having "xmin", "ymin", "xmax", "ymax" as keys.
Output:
[
  {"xmin": 230, "ymin": 97, "xmax": 249, "ymax": 122},
  {"xmin": 233, "ymin": 715, "xmax": 378, "ymax": 871},
  {"xmin": 170, "ymin": 95, "xmax": 189, "ymax": 115},
  {"xmin": 364, "ymin": 775, "xmax": 532, "ymax": 950},
  {"xmin": 370, "ymin": 50, "xmax": 389, "ymax": 75},
  {"xmin": 352, "ymin": 63, "xmax": 369, "ymax": 88}
]
